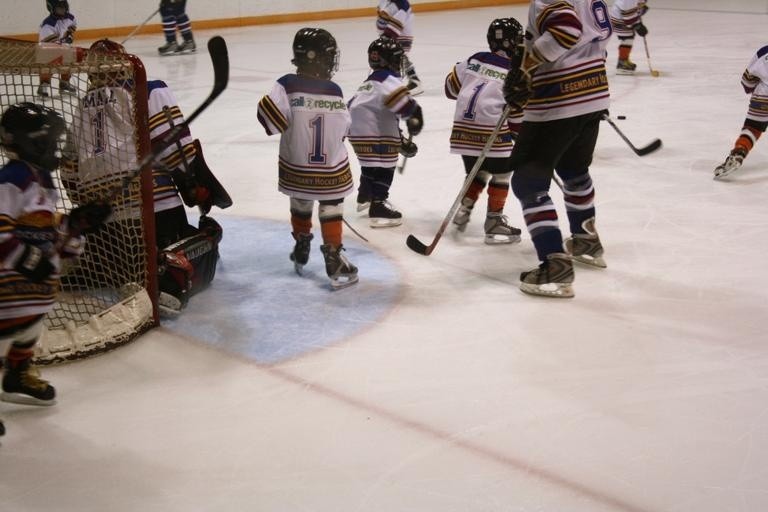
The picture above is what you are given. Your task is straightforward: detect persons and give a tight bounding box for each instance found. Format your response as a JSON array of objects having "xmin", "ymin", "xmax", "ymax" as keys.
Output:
[
  {"xmin": 444, "ymin": 17, "xmax": 527, "ymax": 247},
  {"xmin": 255, "ymin": 26, "xmax": 369, "ymax": 293},
  {"xmin": 37, "ymin": 0, "xmax": 80, "ymax": 100},
  {"xmin": 502, "ymin": 0, "xmax": 613, "ymax": 298},
  {"xmin": 345, "ymin": 34, "xmax": 423, "ymax": 229},
  {"xmin": 58, "ymin": 38, "xmax": 234, "ymax": 320},
  {"xmin": 156, "ymin": 1, "xmax": 197, "ymax": 56},
  {"xmin": 0, "ymin": 99, "xmax": 113, "ymax": 438},
  {"xmin": 713, "ymin": 45, "xmax": 768, "ymax": 181},
  {"xmin": 608, "ymin": 0, "xmax": 650, "ymax": 76},
  {"xmin": 374, "ymin": 1, "xmax": 425, "ymax": 97}
]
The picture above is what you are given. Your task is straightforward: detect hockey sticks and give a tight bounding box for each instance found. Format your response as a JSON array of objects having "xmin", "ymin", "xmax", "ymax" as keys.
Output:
[
  {"xmin": 79, "ymin": 36, "xmax": 231, "ymax": 210},
  {"xmin": 639, "ymin": 14, "xmax": 661, "ymax": 79},
  {"xmin": 604, "ymin": 113, "xmax": 662, "ymax": 158},
  {"xmin": 406, "ymin": 103, "xmax": 514, "ymax": 256}
]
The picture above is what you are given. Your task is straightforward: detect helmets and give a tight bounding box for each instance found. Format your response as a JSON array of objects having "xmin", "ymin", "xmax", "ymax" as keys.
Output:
[
  {"xmin": 46, "ymin": 0, "xmax": 70, "ymax": 17},
  {"xmin": 487, "ymin": 17, "xmax": 525, "ymax": 60},
  {"xmin": 0, "ymin": 102, "xmax": 66, "ymax": 174},
  {"xmin": 87, "ymin": 39, "xmax": 128, "ymax": 85},
  {"xmin": 369, "ymin": 38, "xmax": 409, "ymax": 79},
  {"xmin": 292, "ymin": 28, "xmax": 340, "ymax": 81}
]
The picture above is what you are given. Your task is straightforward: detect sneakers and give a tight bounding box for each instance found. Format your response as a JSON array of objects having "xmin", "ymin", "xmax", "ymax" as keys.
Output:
[
  {"xmin": 157, "ymin": 40, "xmax": 177, "ymax": 54},
  {"xmin": 37, "ymin": 81, "xmax": 50, "ymax": 96},
  {"xmin": 561, "ymin": 236, "xmax": 604, "ymax": 258},
  {"xmin": 1, "ymin": 362, "xmax": 56, "ymax": 400},
  {"xmin": 520, "ymin": 254, "xmax": 576, "ymax": 284},
  {"xmin": 617, "ymin": 58, "xmax": 636, "ymax": 71},
  {"xmin": 289, "ymin": 232, "xmax": 315, "ymax": 265},
  {"xmin": 320, "ymin": 243, "xmax": 359, "ymax": 276},
  {"xmin": 357, "ymin": 192, "xmax": 373, "ymax": 204},
  {"xmin": 368, "ymin": 202, "xmax": 403, "ymax": 221},
  {"xmin": 175, "ymin": 39, "xmax": 196, "ymax": 54},
  {"xmin": 483, "ymin": 210, "xmax": 521, "ymax": 236},
  {"xmin": 714, "ymin": 148, "xmax": 745, "ymax": 176},
  {"xmin": 452, "ymin": 205, "xmax": 472, "ymax": 225},
  {"xmin": 60, "ymin": 79, "xmax": 76, "ymax": 92}
]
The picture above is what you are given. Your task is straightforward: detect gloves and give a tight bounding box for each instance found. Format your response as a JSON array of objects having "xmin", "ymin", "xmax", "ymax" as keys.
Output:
[
  {"xmin": 57, "ymin": 219, "xmax": 85, "ymax": 261},
  {"xmin": 634, "ymin": 22, "xmax": 648, "ymax": 37},
  {"xmin": 407, "ymin": 106, "xmax": 423, "ymax": 135},
  {"xmin": 399, "ymin": 131, "xmax": 417, "ymax": 158},
  {"xmin": 17, "ymin": 245, "xmax": 58, "ymax": 284},
  {"xmin": 503, "ymin": 56, "xmax": 535, "ymax": 111}
]
